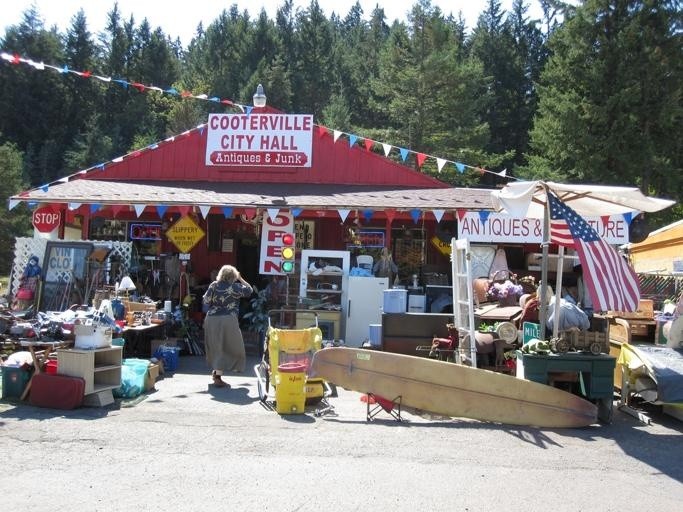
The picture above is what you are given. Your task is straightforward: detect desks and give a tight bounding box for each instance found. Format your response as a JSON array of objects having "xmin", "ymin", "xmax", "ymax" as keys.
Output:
[
  {"xmin": 516, "ymin": 349, "xmax": 615, "ymax": 420},
  {"xmin": 124, "ymin": 322, "xmax": 170, "ymax": 362},
  {"xmin": 294, "ymin": 308, "xmax": 341, "ymax": 346},
  {"xmin": 15, "ymin": 340, "xmax": 71, "ymax": 402}
]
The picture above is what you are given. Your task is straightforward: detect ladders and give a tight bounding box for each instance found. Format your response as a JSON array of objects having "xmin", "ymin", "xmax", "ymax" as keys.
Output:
[{"xmin": 450, "ymin": 236, "xmax": 477, "ymax": 369}]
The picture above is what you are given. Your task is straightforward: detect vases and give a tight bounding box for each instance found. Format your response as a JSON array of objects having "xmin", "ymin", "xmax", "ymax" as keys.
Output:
[{"xmin": 498, "ymin": 293, "xmax": 516, "ymax": 306}]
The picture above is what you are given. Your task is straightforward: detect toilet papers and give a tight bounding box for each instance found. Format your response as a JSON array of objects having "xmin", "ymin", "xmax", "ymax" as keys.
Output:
[{"xmin": 165, "ymin": 300, "xmax": 172, "ymax": 314}]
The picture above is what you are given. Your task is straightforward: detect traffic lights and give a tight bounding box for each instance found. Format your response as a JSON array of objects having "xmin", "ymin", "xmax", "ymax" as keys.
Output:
[{"xmin": 280, "ymin": 232, "xmax": 296, "ymax": 276}]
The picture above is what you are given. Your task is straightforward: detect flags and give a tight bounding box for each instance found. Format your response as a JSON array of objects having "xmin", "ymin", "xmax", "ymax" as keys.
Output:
[{"xmin": 548, "ymin": 191, "xmax": 641, "ymax": 312}]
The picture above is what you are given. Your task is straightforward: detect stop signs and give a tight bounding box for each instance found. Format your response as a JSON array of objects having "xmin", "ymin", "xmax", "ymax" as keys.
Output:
[{"xmin": 32, "ymin": 205, "xmax": 61, "ymax": 233}]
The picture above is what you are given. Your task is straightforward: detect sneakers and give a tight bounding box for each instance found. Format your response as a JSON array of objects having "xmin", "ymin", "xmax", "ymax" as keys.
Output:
[{"xmin": 214, "ymin": 377, "xmax": 231, "ymax": 389}]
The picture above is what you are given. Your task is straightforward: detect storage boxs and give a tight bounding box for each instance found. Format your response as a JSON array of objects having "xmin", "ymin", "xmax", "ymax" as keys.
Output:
[
  {"xmin": 607, "ymin": 295, "xmax": 654, "ymax": 320},
  {"xmin": 0, "ymin": 364, "xmax": 44, "ymax": 403},
  {"xmin": 143, "ymin": 364, "xmax": 158, "ymax": 393}
]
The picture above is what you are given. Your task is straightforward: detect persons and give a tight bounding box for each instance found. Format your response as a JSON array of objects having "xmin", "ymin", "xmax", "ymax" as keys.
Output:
[
  {"xmin": 373, "ymin": 246, "xmax": 399, "ymax": 289},
  {"xmin": 201, "ymin": 264, "xmax": 253, "ymax": 387},
  {"xmin": 534, "ymin": 282, "xmax": 595, "ymax": 334},
  {"xmin": 17, "ymin": 255, "xmax": 41, "ymax": 281}
]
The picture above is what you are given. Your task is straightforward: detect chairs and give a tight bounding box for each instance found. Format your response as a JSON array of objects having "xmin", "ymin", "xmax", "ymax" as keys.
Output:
[{"xmin": 356, "ymin": 254, "xmax": 374, "ymax": 275}]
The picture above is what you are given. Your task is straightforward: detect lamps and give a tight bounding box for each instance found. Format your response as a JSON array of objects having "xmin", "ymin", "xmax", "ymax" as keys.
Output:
[{"xmin": 115, "ymin": 275, "xmax": 137, "ymax": 297}]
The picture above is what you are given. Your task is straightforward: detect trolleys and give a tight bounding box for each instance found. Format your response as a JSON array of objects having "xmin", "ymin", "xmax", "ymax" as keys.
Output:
[{"xmin": 252, "ymin": 308, "xmax": 333, "ymax": 417}]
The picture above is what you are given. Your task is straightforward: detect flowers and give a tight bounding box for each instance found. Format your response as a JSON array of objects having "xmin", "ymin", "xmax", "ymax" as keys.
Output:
[{"xmin": 487, "ymin": 280, "xmax": 522, "ymax": 298}]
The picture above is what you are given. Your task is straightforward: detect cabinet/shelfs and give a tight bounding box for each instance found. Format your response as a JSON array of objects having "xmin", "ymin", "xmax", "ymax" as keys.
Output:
[
  {"xmin": 343, "ymin": 276, "xmax": 390, "ymax": 349},
  {"xmin": 53, "ymin": 345, "xmax": 122, "ymax": 407},
  {"xmin": 298, "ymin": 248, "xmax": 350, "ymax": 344}
]
